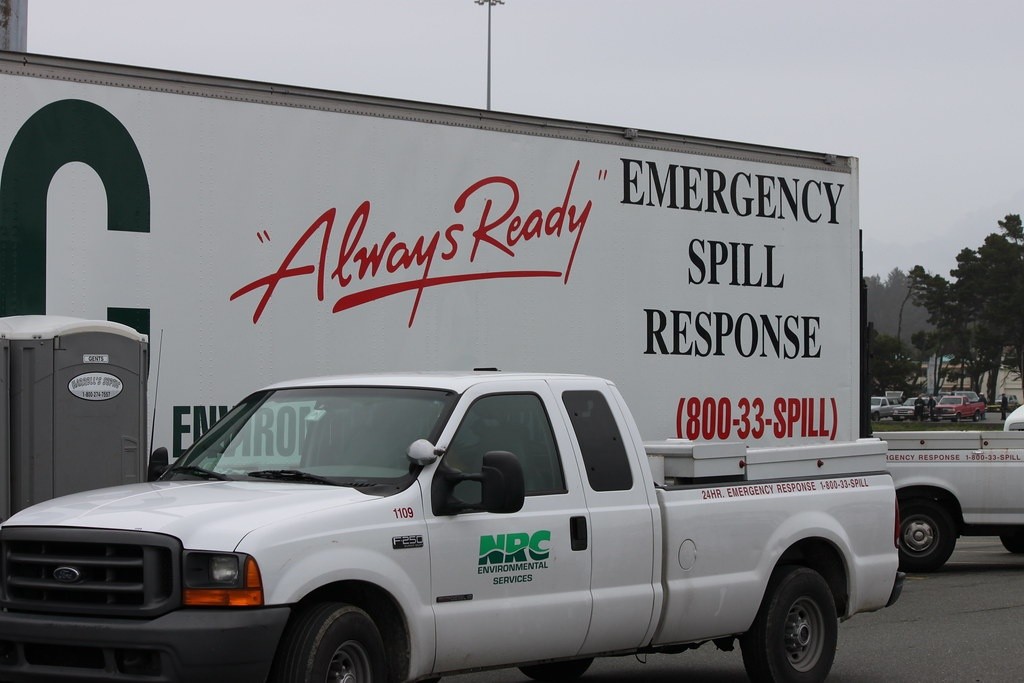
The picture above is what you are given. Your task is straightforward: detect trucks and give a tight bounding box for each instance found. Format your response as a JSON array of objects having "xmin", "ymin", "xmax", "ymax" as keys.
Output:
[{"xmin": 870, "ymin": 397, "xmax": 902, "ymax": 421}]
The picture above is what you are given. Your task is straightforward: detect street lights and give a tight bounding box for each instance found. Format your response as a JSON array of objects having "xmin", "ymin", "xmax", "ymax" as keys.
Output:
[{"xmin": 475, "ymin": 0, "xmax": 505, "ymax": 112}]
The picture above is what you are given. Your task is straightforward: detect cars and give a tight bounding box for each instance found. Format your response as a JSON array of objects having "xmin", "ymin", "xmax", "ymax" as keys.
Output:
[
  {"xmin": 951, "ymin": 391, "xmax": 979, "ymax": 401},
  {"xmin": 890, "ymin": 397, "xmax": 939, "ymax": 421},
  {"xmin": 995, "ymin": 394, "xmax": 1018, "ymax": 403}
]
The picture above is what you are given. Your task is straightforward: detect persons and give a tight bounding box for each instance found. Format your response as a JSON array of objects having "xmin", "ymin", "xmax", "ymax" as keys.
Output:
[
  {"xmin": 978, "ymin": 392, "xmax": 986, "ymax": 406},
  {"xmin": 914, "ymin": 395, "xmax": 924, "ymax": 421},
  {"xmin": 1000, "ymin": 393, "xmax": 1008, "ymax": 420},
  {"xmin": 927, "ymin": 395, "xmax": 936, "ymax": 422}
]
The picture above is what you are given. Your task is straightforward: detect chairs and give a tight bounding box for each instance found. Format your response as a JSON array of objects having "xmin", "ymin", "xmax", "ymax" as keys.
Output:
[{"xmin": 455, "ymin": 408, "xmax": 541, "ymax": 504}]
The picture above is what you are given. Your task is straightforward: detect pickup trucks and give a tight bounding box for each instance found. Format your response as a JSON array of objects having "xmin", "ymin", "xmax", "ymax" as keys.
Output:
[
  {"xmin": 872, "ymin": 403, "xmax": 1024, "ymax": 574},
  {"xmin": 934, "ymin": 396, "xmax": 985, "ymax": 422},
  {"xmin": 0, "ymin": 367, "xmax": 907, "ymax": 683}
]
